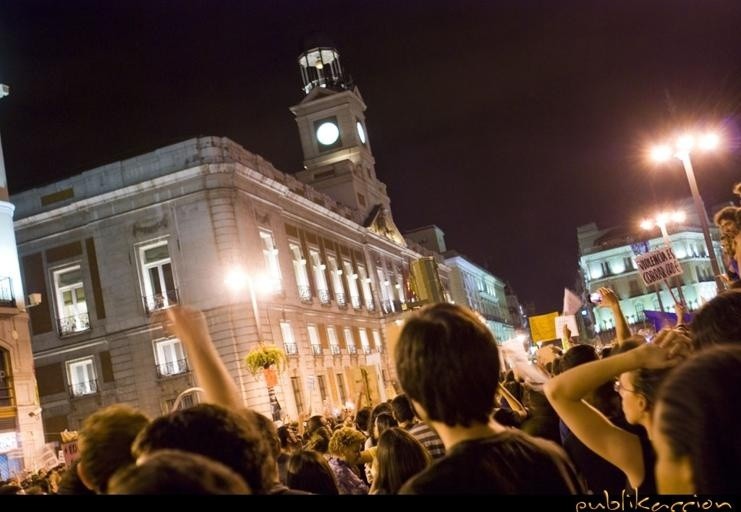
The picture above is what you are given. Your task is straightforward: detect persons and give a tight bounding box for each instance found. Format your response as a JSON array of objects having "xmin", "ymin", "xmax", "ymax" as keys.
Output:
[{"xmin": 0, "ymin": 184, "xmax": 741, "ymax": 512}]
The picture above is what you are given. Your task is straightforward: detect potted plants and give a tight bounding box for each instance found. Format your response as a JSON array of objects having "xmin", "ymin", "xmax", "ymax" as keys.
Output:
[{"xmin": 245, "ymin": 344, "xmax": 288, "ymax": 387}]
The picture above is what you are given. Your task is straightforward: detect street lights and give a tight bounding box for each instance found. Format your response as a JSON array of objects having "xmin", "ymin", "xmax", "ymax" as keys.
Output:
[
  {"xmin": 637, "ymin": 181, "xmax": 691, "ymax": 315},
  {"xmin": 224, "ymin": 271, "xmax": 282, "ymax": 428},
  {"xmin": 645, "ymin": 87, "xmax": 731, "ymax": 294}
]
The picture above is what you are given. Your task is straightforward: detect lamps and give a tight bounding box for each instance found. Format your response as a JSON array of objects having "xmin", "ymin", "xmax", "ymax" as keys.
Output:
[
  {"xmin": 20, "ymin": 292, "xmax": 43, "ymax": 314},
  {"xmin": 315, "ymin": 56, "xmax": 324, "ymax": 69}
]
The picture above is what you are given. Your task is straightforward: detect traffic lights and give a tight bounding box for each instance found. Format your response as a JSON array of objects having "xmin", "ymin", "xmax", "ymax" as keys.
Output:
[{"xmin": 581, "ymin": 308, "xmax": 591, "ymax": 326}]
